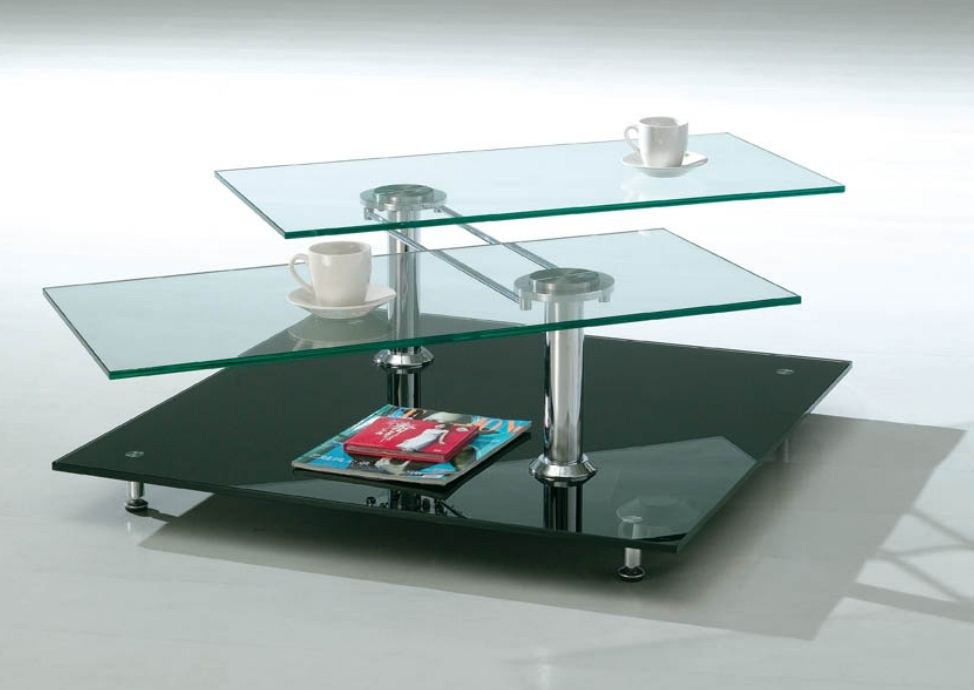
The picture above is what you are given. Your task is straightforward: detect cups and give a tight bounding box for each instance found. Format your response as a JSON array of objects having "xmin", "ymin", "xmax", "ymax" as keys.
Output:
[
  {"xmin": 287, "ymin": 241, "xmax": 372, "ymax": 307},
  {"xmin": 623, "ymin": 117, "xmax": 690, "ymax": 166}
]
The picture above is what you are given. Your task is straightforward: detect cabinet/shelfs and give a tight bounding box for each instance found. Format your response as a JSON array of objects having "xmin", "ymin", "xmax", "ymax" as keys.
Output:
[{"xmin": 43, "ymin": 135, "xmax": 856, "ymax": 580}]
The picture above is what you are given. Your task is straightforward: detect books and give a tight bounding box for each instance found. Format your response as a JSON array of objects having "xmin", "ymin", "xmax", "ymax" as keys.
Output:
[
  {"xmin": 343, "ymin": 416, "xmax": 480, "ymax": 464},
  {"xmin": 291, "ymin": 404, "xmax": 533, "ymax": 488}
]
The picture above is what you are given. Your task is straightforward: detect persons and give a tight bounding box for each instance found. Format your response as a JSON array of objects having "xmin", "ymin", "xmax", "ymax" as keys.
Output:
[{"xmin": 397, "ymin": 422, "xmax": 450, "ymax": 450}]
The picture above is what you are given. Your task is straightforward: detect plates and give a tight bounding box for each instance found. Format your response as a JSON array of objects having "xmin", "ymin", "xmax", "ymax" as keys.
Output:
[
  {"xmin": 287, "ymin": 282, "xmax": 398, "ymax": 321},
  {"xmin": 622, "ymin": 149, "xmax": 713, "ymax": 177}
]
